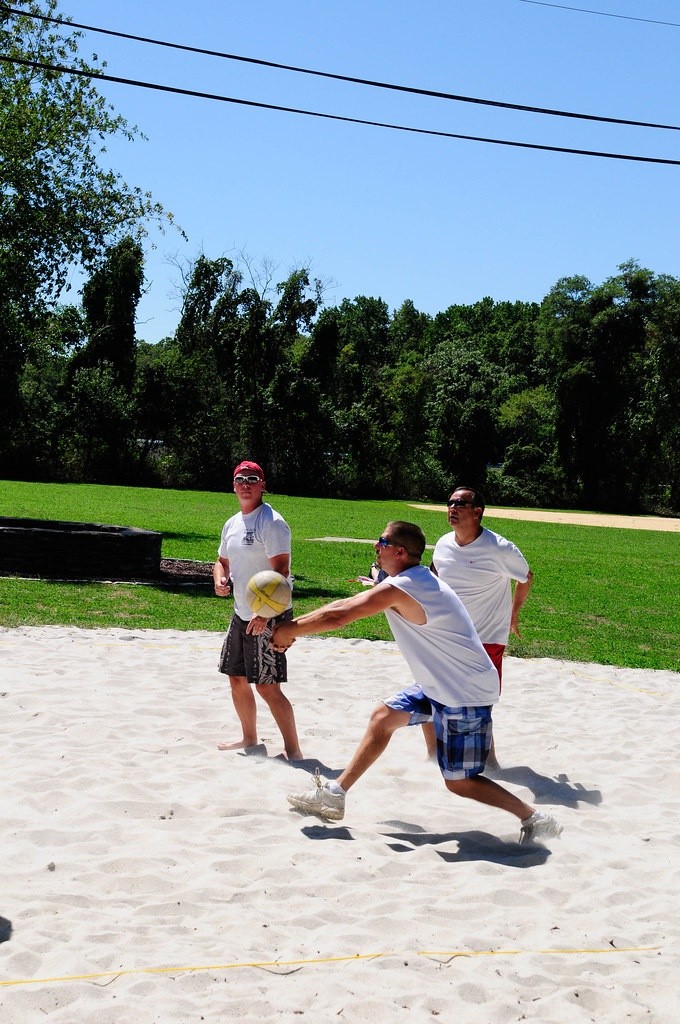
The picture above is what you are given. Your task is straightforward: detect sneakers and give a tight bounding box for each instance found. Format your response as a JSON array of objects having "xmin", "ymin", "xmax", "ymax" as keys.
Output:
[
  {"xmin": 519, "ymin": 814, "xmax": 564, "ymax": 849},
  {"xmin": 286, "ymin": 767, "xmax": 345, "ymax": 819}
]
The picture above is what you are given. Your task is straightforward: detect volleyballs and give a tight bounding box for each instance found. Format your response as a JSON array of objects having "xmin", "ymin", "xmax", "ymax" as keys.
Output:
[{"xmin": 244, "ymin": 570, "xmax": 292, "ymax": 618}]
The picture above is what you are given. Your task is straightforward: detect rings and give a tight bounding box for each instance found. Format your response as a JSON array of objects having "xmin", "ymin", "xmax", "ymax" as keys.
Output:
[{"xmin": 259, "ymin": 628, "xmax": 261, "ymax": 629}]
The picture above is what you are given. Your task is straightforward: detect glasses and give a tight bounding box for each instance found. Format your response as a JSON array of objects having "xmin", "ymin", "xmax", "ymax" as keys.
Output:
[
  {"xmin": 233, "ymin": 475, "xmax": 262, "ymax": 484},
  {"xmin": 447, "ymin": 499, "xmax": 478, "ymax": 507},
  {"xmin": 379, "ymin": 537, "xmax": 407, "ymax": 550}
]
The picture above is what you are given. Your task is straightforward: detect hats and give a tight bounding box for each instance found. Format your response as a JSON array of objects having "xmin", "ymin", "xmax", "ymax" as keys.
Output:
[{"xmin": 234, "ymin": 460, "xmax": 265, "ymax": 477}]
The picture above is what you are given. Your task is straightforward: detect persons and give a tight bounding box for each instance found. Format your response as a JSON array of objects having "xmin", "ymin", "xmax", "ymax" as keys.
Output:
[
  {"xmin": 267, "ymin": 521, "xmax": 564, "ymax": 846},
  {"xmin": 214, "ymin": 461, "xmax": 303, "ymax": 761},
  {"xmin": 421, "ymin": 485, "xmax": 534, "ymax": 767}
]
